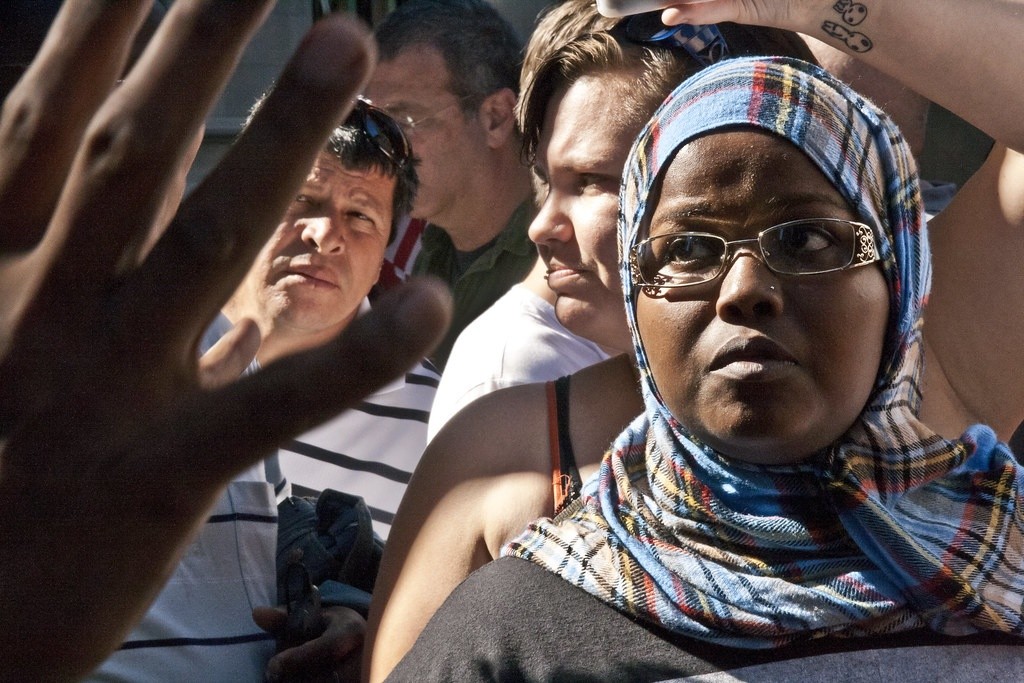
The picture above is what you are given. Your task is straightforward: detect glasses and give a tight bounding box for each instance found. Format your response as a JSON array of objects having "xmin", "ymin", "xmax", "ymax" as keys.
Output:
[
  {"xmin": 381, "ymin": 88, "xmax": 495, "ymax": 138},
  {"xmin": 622, "ymin": 12, "xmax": 732, "ymax": 69},
  {"xmin": 629, "ymin": 219, "xmax": 881, "ymax": 289}
]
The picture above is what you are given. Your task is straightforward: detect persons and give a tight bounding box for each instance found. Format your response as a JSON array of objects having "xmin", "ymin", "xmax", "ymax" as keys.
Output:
[
  {"xmin": 0, "ymin": 1, "xmax": 457, "ymax": 683},
  {"xmin": 371, "ymin": 58, "xmax": 1024, "ymax": 683},
  {"xmin": 207, "ymin": 88, "xmax": 442, "ymax": 596},
  {"xmin": 349, "ymin": 0, "xmax": 550, "ymax": 379},
  {"xmin": 0, "ymin": 0, "xmax": 372, "ymax": 683},
  {"xmin": 362, "ymin": 0, "xmax": 1024, "ymax": 683}
]
